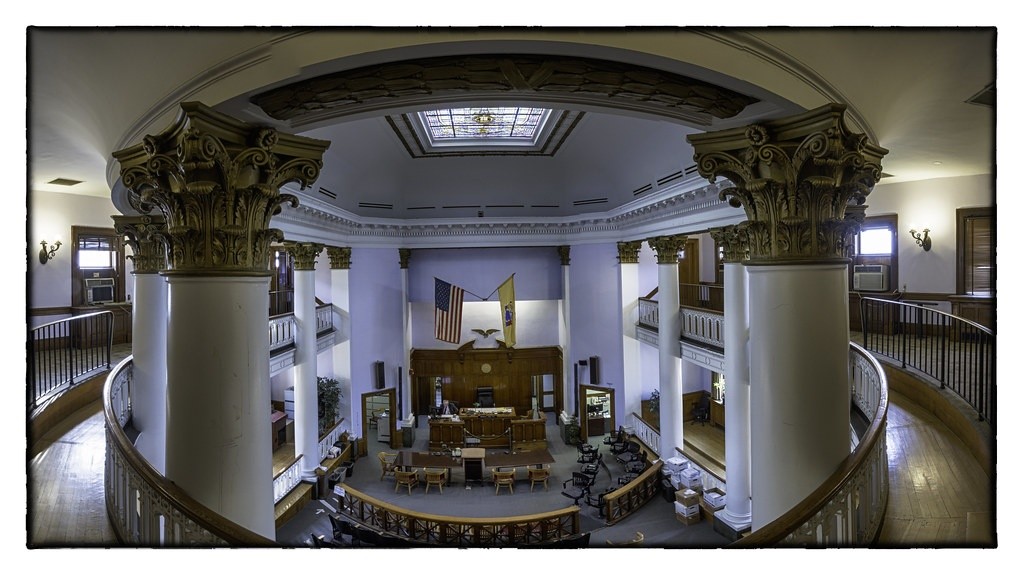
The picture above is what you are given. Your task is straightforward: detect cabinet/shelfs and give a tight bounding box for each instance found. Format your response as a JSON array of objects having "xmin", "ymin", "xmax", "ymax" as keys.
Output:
[{"xmin": 69, "ymin": 300, "xmax": 134, "ymax": 350}]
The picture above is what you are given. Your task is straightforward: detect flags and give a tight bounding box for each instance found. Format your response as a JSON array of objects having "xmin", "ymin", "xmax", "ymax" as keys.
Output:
[
  {"xmin": 496, "ymin": 275, "xmax": 517, "ymax": 349},
  {"xmin": 432, "ymin": 278, "xmax": 465, "ymax": 344}
]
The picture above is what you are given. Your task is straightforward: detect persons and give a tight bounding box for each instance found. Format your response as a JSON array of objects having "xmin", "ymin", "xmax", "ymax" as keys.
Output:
[{"xmin": 436, "ymin": 399, "xmax": 458, "ymax": 416}]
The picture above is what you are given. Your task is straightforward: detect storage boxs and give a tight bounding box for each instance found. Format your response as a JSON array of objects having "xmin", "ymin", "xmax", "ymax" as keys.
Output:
[{"xmin": 661, "ymin": 456, "xmax": 727, "ymax": 527}]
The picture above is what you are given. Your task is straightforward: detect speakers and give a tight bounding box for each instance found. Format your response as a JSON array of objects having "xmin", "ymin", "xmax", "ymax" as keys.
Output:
[
  {"xmin": 589, "ymin": 356, "xmax": 599, "ymax": 384},
  {"xmin": 376, "ymin": 361, "xmax": 386, "ymax": 389}
]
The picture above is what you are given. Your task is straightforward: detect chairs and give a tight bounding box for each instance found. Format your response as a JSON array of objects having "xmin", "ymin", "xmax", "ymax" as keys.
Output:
[
  {"xmin": 557, "ymin": 425, "xmax": 649, "ymax": 511},
  {"xmin": 528, "ymin": 468, "xmax": 549, "ymax": 491},
  {"xmin": 423, "ymin": 467, "xmax": 448, "ymax": 494},
  {"xmin": 378, "ymin": 451, "xmax": 396, "ymax": 481},
  {"xmin": 492, "ymin": 468, "xmax": 516, "ymax": 494},
  {"xmin": 394, "ymin": 466, "xmax": 420, "ymax": 495}
]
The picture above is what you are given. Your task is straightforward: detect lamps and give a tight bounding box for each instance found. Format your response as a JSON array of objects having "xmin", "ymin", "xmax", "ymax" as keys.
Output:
[
  {"xmin": 908, "ymin": 228, "xmax": 931, "ymax": 251},
  {"xmin": 39, "ymin": 240, "xmax": 63, "ymax": 265}
]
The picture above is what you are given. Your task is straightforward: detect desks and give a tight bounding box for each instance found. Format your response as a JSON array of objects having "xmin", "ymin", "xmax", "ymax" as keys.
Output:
[{"xmin": 394, "ymin": 447, "xmax": 555, "ymax": 478}]
